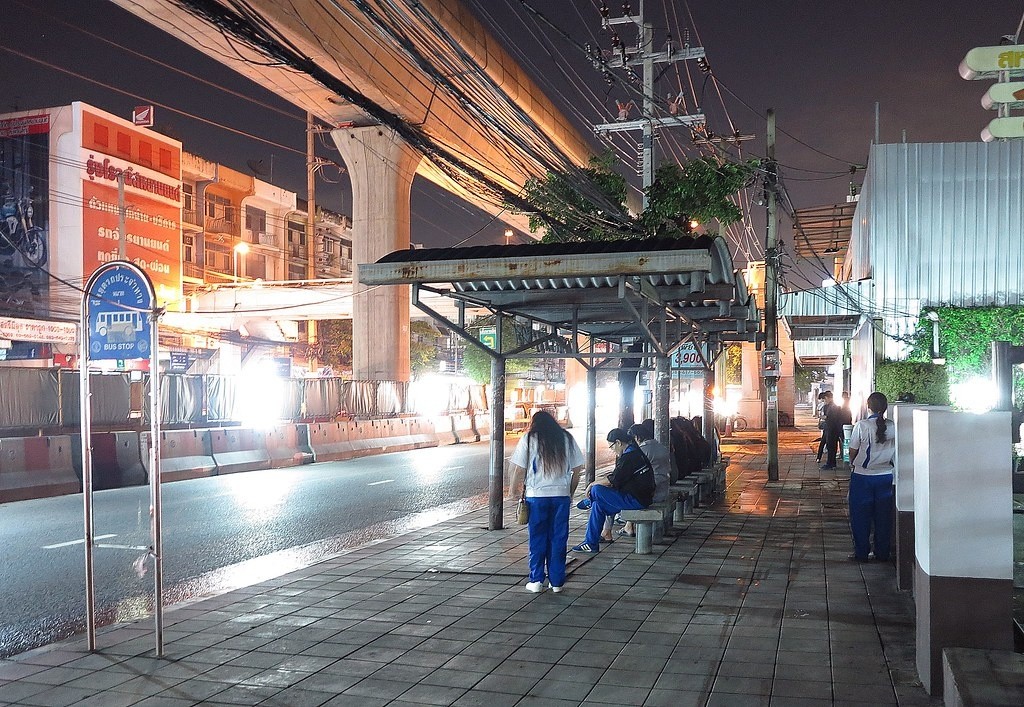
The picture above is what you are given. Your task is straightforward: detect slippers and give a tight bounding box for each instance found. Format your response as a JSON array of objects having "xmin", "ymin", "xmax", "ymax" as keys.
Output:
[
  {"xmin": 599, "ymin": 536, "xmax": 615, "ymax": 544},
  {"xmin": 615, "ymin": 527, "xmax": 636, "ymax": 540}
]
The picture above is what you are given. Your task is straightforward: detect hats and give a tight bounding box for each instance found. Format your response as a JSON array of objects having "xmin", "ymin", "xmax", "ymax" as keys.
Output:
[
  {"xmin": 822, "ymin": 391, "xmax": 833, "ymax": 398},
  {"xmin": 818, "ymin": 392, "xmax": 827, "ymax": 399}
]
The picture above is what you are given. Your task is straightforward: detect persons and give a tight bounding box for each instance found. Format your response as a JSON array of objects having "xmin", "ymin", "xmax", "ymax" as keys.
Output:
[
  {"xmin": 509, "ymin": 411, "xmax": 584, "ymax": 592},
  {"xmin": 614, "ymin": 419, "xmax": 678, "ymax": 526},
  {"xmin": 816, "ymin": 390, "xmax": 852, "ymax": 470},
  {"xmin": 598, "ymin": 423, "xmax": 672, "ymax": 543},
  {"xmin": 570, "ymin": 428, "xmax": 656, "ymax": 553},
  {"xmin": 849, "ymin": 392, "xmax": 895, "ymax": 562},
  {"xmin": 671, "ymin": 415, "xmax": 723, "ymax": 483}
]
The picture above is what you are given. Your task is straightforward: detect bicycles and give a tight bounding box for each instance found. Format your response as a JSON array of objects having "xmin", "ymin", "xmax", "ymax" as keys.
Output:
[
  {"xmin": 731, "ymin": 412, "xmax": 747, "ymax": 432},
  {"xmin": 778, "ymin": 411, "xmax": 790, "ymax": 426}
]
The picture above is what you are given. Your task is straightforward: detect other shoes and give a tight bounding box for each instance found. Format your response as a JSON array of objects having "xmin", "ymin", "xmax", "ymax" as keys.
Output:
[
  {"xmin": 845, "ymin": 551, "xmax": 892, "ymax": 563},
  {"xmin": 815, "ymin": 459, "xmax": 822, "ymax": 463},
  {"xmin": 818, "ymin": 464, "xmax": 836, "ymax": 470},
  {"xmin": 836, "ymin": 453, "xmax": 844, "ymax": 461}
]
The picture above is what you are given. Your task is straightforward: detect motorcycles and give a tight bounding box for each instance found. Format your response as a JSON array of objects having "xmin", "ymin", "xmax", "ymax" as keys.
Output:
[{"xmin": 0, "ymin": 186, "xmax": 48, "ymax": 270}]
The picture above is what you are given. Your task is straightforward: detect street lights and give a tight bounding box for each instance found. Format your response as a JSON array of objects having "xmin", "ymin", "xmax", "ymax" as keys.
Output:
[
  {"xmin": 233, "ymin": 242, "xmax": 249, "ymax": 310},
  {"xmin": 504, "ymin": 229, "xmax": 514, "ymax": 244},
  {"xmin": 116, "ymin": 169, "xmax": 137, "ymax": 261}
]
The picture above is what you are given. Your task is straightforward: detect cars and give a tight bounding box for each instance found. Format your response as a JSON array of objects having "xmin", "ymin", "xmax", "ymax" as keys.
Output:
[{"xmin": 505, "ymin": 372, "xmax": 527, "ymax": 388}]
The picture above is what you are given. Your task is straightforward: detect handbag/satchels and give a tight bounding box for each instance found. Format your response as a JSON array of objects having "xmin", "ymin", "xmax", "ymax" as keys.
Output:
[{"xmin": 513, "ymin": 497, "xmax": 528, "ymax": 525}]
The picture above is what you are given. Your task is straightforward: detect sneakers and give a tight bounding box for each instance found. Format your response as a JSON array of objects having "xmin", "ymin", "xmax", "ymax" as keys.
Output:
[
  {"xmin": 548, "ymin": 583, "xmax": 562, "ymax": 592},
  {"xmin": 571, "ymin": 539, "xmax": 600, "ymax": 553},
  {"xmin": 525, "ymin": 581, "xmax": 543, "ymax": 594},
  {"xmin": 576, "ymin": 498, "xmax": 593, "ymax": 512},
  {"xmin": 614, "ymin": 516, "xmax": 627, "ymax": 527}
]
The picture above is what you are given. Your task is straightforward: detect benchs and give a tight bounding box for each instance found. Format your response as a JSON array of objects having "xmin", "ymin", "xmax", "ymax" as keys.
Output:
[{"xmin": 621, "ymin": 456, "xmax": 731, "ymax": 554}]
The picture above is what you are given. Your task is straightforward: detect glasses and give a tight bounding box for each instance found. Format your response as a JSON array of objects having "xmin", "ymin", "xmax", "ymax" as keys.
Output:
[
  {"xmin": 818, "ymin": 397, "xmax": 825, "ymax": 400},
  {"xmin": 608, "ymin": 442, "xmax": 617, "ymax": 450}
]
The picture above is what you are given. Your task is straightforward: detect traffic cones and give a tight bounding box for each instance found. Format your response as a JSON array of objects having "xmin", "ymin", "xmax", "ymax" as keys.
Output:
[{"xmin": 721, "ymin": 418, "xmax": 737, "ymax": 438}]
